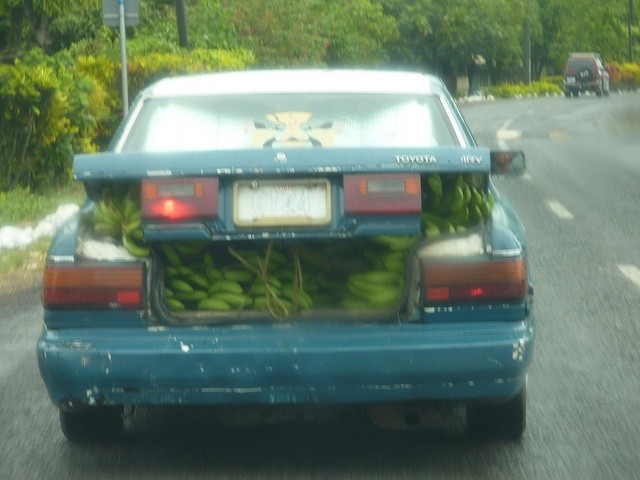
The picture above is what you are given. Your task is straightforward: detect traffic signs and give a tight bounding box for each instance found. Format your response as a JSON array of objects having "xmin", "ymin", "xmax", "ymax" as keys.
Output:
[{"xmin": 103, "ymin": 1, "xmax": 140, "ymax": 27}]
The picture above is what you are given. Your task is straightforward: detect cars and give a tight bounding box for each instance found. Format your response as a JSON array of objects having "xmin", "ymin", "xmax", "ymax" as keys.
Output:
[{"xmin": 37, "ymin": 69, "xmax": 533, "ymax": 441}]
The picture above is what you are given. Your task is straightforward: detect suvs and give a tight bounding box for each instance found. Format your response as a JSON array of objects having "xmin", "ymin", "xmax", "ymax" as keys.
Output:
[{"xmin": 564, "ymin": 52, "xmax": 609, "ymax": 97}]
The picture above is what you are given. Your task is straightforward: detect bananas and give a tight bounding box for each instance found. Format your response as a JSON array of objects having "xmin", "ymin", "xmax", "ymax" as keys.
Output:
[{"xmin": 92, "ymin": 172, "xmax": 495, "ymax": 315}]
